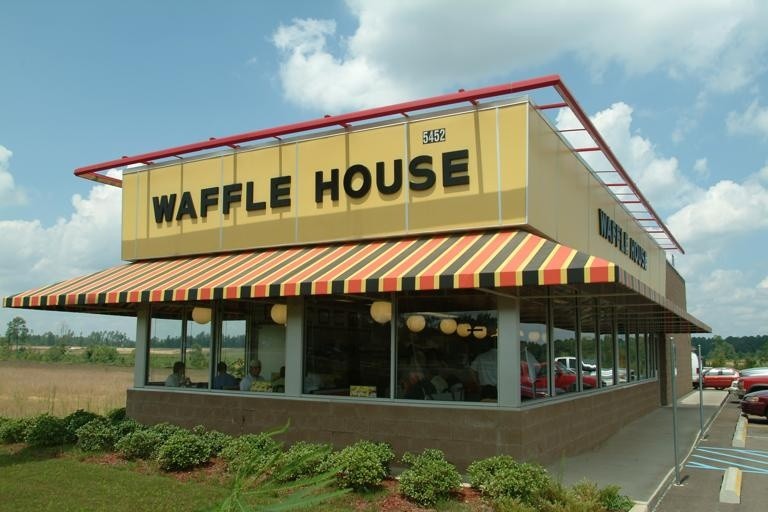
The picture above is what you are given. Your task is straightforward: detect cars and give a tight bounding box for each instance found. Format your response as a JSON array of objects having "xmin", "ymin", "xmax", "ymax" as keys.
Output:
[
  {"xmin": 521, "ymin": 355, "xmax": 631, "ymax": 401},
  {"xmin": 689, "ymin": 351, "xmax": 768, "ymax": 419}
]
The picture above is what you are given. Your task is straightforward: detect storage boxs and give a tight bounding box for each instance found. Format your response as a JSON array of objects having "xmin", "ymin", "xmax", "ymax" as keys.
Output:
[
  {"xmin": 349, "ymin": 384, "xmax": 377, "ymax": 398},
  {"xmin": 249, "ymin": 379, "xmax": 273, "ymax": 392}
]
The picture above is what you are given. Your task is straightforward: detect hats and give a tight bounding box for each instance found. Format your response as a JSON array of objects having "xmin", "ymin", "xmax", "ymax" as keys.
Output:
[{"xmin": 249, "ymin": 359, "xmax": 262, "ymax": 368}]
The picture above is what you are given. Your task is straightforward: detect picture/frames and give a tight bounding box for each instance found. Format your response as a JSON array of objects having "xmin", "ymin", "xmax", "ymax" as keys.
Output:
[{"xmin": 308, "ymin": 307, "xmax": 370, "ymax": 328}]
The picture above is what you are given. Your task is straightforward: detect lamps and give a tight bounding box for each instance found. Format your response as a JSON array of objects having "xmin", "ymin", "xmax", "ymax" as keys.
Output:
[
  {"xmin": 371, "ymin": 298, "xmax": 542, "ymax": 344},
  {"xmin": 269, "ymin": 297, "xmax": 286, "ymax": 324},
  {"xmin": 191, "ymin": 297, "xmax": 211, "ymax": 326}
]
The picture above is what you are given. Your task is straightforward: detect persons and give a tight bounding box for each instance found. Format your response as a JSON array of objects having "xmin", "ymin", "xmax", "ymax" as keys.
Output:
[
  {"xmin": 470, "ymin": 338, "xmax": 497, "ymax": 399},
  {"xmin": 240, "ymin": 359, "xmax": 264, "ymax": 391},
  {"xmin": 165, "ymin": 361, "xmax": 190, "ymax": 388},
  {"xmin": 272, "ymin": 366, "xmax": 285, "ymax": 386},
  {"xmin": 212, "ymin": 361, "xmax": 237, "ymax": 390}
]
